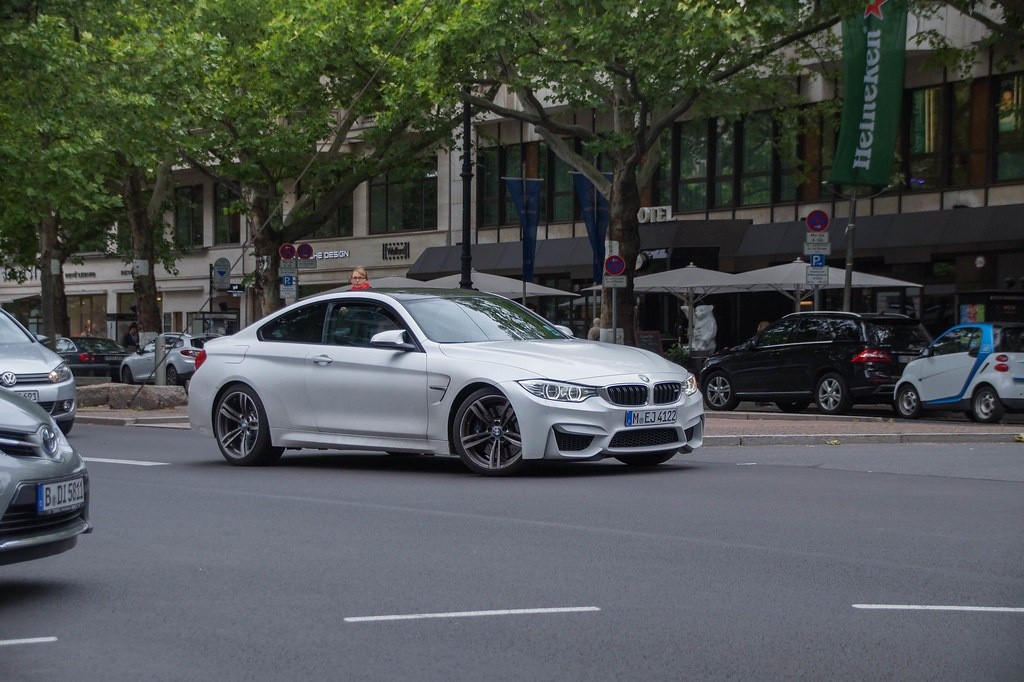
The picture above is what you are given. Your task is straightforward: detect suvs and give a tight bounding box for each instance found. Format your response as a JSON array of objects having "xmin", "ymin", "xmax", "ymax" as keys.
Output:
[{"xmin": 697, "ymin": 310, "xmax": 938, "ymax": 416}]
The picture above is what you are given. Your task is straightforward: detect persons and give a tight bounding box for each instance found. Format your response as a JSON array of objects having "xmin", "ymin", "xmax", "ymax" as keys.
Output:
[
  {"xmin": 339, "ymin": 266, "xmax": 375, "ymax": 315},
  {"xmin": 224, "ymin": 319, "xmax": 234, "ymax": 335},
  {"xmin": 586, "ymin": 317, "xmax": 606, "ymax": 344},
  {"xmin": 1000, "ymin": 85, "xmax": 1015, "ymax": 118},
  {"xmin": 81, "ymin": 326, "xmax": 93, "ymax": 338},
  {"xmin": 755, "ymin": 319, "xmax": 774, "ymax": 406},
  {"xmin": 122, "ymin": 322, "xmax": 142, "ymax": 350}
]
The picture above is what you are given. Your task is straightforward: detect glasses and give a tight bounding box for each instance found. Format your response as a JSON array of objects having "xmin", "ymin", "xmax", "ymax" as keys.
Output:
[{"xmin": 352, "ymin": 277, "xmax": 365, "ymax": 281}]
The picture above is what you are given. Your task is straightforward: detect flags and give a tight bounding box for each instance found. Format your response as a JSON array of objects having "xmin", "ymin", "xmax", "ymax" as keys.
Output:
[{"xmin": 835, "ymin": 0, "xmax": 908, "ymax": 186}]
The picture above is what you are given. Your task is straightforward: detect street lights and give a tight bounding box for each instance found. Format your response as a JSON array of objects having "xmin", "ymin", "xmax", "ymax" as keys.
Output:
[{"xmin": 449, "ymin": 68, "xmax": 481, "ymax": 293}]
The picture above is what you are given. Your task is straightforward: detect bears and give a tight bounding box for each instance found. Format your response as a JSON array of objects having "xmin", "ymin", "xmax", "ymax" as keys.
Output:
[{"xmin": 680, "ymin": 304, "xmax": 717, "ymax": 357}]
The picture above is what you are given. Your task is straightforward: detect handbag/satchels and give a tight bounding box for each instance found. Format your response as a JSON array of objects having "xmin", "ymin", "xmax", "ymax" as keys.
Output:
[{"xmin": 333, "ymin": 311, "xmax": 350, "ymax": 337}]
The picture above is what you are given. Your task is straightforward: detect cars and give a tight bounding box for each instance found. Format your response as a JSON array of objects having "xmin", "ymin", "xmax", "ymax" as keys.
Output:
[
  {"xmin": 119, "ymin": 330, "xmax": 228, "ymax": 388},
  {"xmin": 186, "ymin": 286, "xmax": 707, "ymax": 475},
  {"xmin": 0, "ymin": 389, "xmax": 94, "ymax": 564},
  {"xmin": 0, "ymin": 306, "xmax": 79, "ymax": 435},
  {"xmin": 36, "ymin": 335, "xmax": 132, "ymax": 385},
  {"xmin": 892, "ymin": 320, "xmax": 1024, "ymax": 425}
]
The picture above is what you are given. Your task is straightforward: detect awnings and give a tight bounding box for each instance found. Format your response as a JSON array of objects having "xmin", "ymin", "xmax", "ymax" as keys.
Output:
[
  {"xmin": 405, "ymin": 236, "xmax": 603, "ymax": 280},
  {"xmin": 0, "ymin": 293, "xmax": 40, "ymax": 304}
]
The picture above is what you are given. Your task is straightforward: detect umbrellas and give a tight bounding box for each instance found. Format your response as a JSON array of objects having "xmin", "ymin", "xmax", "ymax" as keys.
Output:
[
  {"xmin": 297, "ymin": 268, "xmax": 581, "ymax": 302},
  {"xmin": 581, "ymin": 257, "xmax": 923, "ymax": 352}
]
